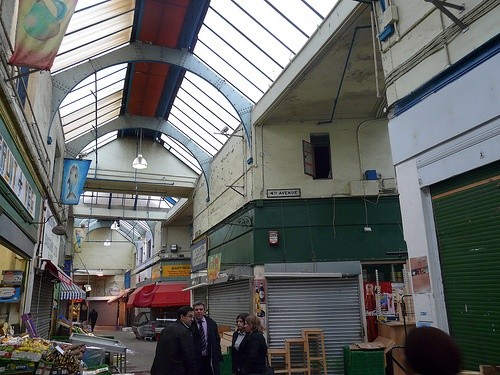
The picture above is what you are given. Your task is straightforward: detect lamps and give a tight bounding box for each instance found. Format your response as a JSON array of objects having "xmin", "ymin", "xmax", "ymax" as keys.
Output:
[
  {"xmin": 28, "ymin": 215, "xmax": 65, "ymax": 235},
  {"xmin": 103, "ymin": 235, "xmax": 111, "ymax": 246},
  {"xmin": 170, "ymin": 244, "xmax": 178, "ymax": 254},
  {"xmin": 110, "ymin": 220, "xmax": 119, "ymax": 230},
  {"xmin": 131, "ymin": 129, "xmax": 148, "ymax": 169}
]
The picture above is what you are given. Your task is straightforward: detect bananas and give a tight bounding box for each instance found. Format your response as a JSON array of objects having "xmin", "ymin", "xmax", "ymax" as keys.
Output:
[{"xmin": 21, "ymin": 339, "xmax": 49, "ymax": 351}]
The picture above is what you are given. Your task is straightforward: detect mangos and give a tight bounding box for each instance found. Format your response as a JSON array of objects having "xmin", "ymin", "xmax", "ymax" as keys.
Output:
[
  {"xmin": 0, "ymin": 346, "xmax": 15, "ymax": 352},
  {"xmin": 14, "ymin": 347, "xmax": 42, "ymax": 354}
]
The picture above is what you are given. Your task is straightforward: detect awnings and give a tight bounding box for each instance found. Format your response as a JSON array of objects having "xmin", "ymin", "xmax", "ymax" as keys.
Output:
[
  {"xmin": 107, "ymin": 288, "xmax": 135, "ymax": 304},
  {"xmin": 54, "ymin": 281, "xmax": 86, "ymax": 300},
  {"xmin": 42, "ymin": 258, "xmax": 72, "ymax": 286},
  {"xmin": 126, "ymin": 283, "xmax": 190, "ymax": 308}
]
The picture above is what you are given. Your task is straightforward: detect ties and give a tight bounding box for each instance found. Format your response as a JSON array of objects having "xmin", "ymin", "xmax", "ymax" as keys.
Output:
[{"xmin": 197, "ymin": 320, "xmax": 206, "ymax": 351}]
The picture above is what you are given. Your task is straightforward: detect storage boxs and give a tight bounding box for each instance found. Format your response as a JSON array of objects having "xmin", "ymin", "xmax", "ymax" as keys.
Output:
[
  {"xmin": 0, "ymin": 287, "xmax": 21, "ymax": 303},
  {"xmin": 342, "ymin": 344, "xmax": 384, "ymax": 375},
  {"xmin": 378, "ymin": 321, "xmax": 415, "ymax": 375},
  {"xmin": 2, "ymin": 270, "xmax": 23, "ymax": 285},
  {"xmin": 0, "ymin": 345, "xmax": 110, "ymax": 375}
]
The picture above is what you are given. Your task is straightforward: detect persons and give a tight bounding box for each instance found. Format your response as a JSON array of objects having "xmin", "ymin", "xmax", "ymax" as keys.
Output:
[
  {"xmin": 151, "ymin": 306, "xmax": 207, "ymax": 375},
  {"xmin": 88, "ymin": 307, "xmax": 98, "ymax": 333},
  {"xmin": 403, "ymin": 326, "xmax": 460, "ymax": 375},
  {"xmin": 190, "ymin": 302, "xmax": 225, "ymax": 375},
  {"xmin": 241, "ymin": 315, "xmax": 268, "ymax": 375},
  {"xmin": 231, "ymin": 313, "xmax": 249, "ymax": 375}
]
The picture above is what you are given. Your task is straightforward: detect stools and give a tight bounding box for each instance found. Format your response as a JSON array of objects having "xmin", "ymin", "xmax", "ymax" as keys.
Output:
[
  {"xmin": 267, "ymin": 348, "xmax": 289, "ymax": 375},
  {"xmin": 303, "ymin": 328, "xmax": 327, "ymax": 375},
  {"xmin": 284, "ymin": 338, "xmax": 308, "ymax": 375}
]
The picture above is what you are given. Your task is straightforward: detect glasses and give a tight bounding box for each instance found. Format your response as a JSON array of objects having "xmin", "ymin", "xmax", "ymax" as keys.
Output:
[{"xmin": 184, "ymin": 315, "xmax": 194, "ymax": 319}]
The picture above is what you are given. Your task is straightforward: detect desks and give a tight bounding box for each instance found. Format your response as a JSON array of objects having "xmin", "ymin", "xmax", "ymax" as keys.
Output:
[{"xmin": 69, "ymin": 333, "xmax": 127, "ymax": 373}]
